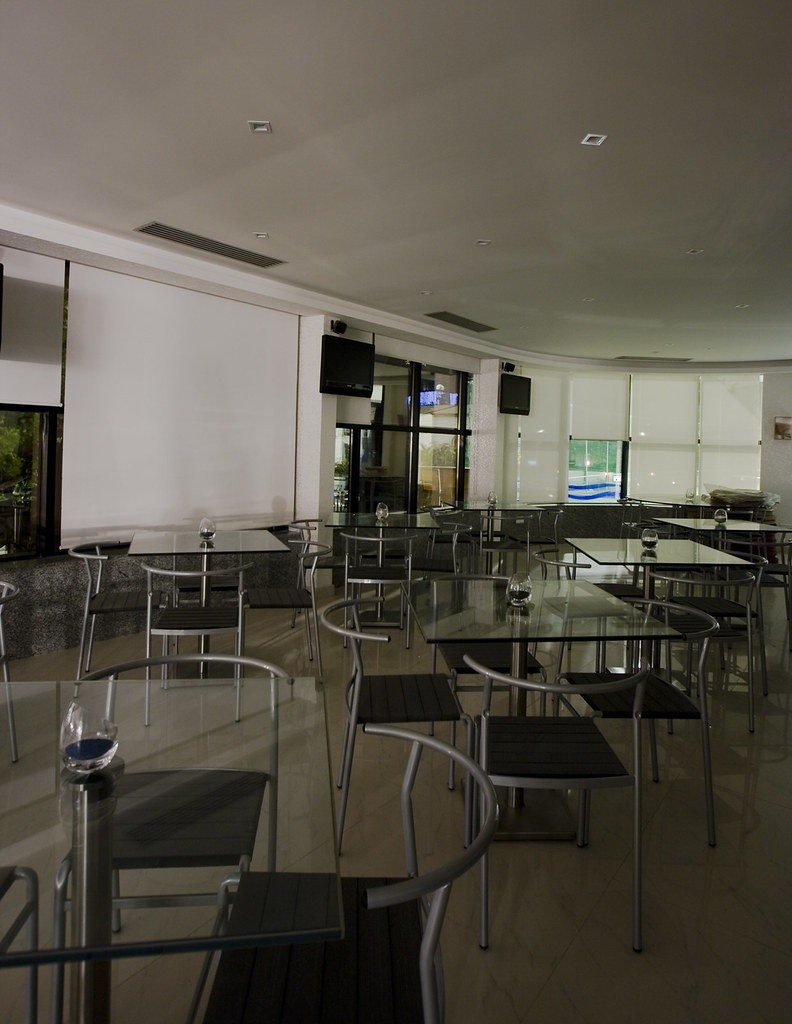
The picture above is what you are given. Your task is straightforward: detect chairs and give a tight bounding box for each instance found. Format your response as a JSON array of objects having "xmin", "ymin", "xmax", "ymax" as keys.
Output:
[{"xmin": 0, "ymin": 497, "xmax": 792, "ymax": 1024}]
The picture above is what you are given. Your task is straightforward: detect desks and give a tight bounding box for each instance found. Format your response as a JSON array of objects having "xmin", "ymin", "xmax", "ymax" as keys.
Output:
[
  {"xmin": 440, "ymin": 499, "xmax": 548, "ymax": 580},
  {"xmin": 650, "ymin": 518, "xmax": 792, "ymax": 630},
  {"xmin": 563, "ymin": 538, "xmax": 757, "ymax": 693},
  {"xmin": 398, "ymin": 579, "xmax": 683, "ymax": 846},
  {"xmin": 127, "ymin": 530, "xmax": 289, "ymax": 728},
  {"xmin": 625, "ymin": 493, "xmax": 765, "ymax": 540},
  {"xmin": 324, "ymin": 511, "xmax": 441, "ymax": 629},
  {"xmin": 0, "ymin": 676, "xmax": 344, "ymax": 1024}
]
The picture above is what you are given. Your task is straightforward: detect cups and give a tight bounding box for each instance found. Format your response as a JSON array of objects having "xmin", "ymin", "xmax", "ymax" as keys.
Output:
[
  {"xmin": 198, "ymin": 516, "xmax": 216, "ymax": 541},
  {"xmin": 714, "ymin": 509, "xmax": 727, "ymax": 523},
  {"xmin": 375, "ymin": 502, "xmax": 388, "ymax": 521},
  {"xmin": 686, "ymin": 489, "xmax": 695, "ymax": 500},
  {"xmin": 58, "ymin": 700, "xmax": 120, "ymax": 775},
  {"xmin": 505, "ymin": 573, "xmax": 532, "ymax": 606},
  {"xmin": 641, "ymin": 528, "xmax": 658, "ymax": 550},
  {"xmin": 488, "ymin": 492, "xmax": 496, "ymax": 504}
]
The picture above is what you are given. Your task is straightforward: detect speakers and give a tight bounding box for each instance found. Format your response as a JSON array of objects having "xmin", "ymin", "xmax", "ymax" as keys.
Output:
[
  {"xmin": 332, "ymin": 320, "xmax": 347, "ymax": 334},
  {"xmin": 504, "ymin": 362, "xmax": 515, "ymax": 372}
]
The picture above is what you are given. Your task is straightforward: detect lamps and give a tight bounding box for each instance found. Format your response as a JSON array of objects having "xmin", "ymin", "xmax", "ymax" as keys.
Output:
[
  {"xmin": 501, "ymin": 361, "xmax": 516, "ymax": 372},
  {"xmin": 330, "ymin": 318, "xmax": 347, "ymax": 335}
]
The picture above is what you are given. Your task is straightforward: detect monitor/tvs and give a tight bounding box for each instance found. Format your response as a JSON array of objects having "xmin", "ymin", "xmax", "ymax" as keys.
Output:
[
  {"xmin": 319, "ymin": 334, "xmax": 375, "ymax": 399},
  {"xmin": 500, "ymin": 373, "xmax": 532, "ymax": 415}
]
